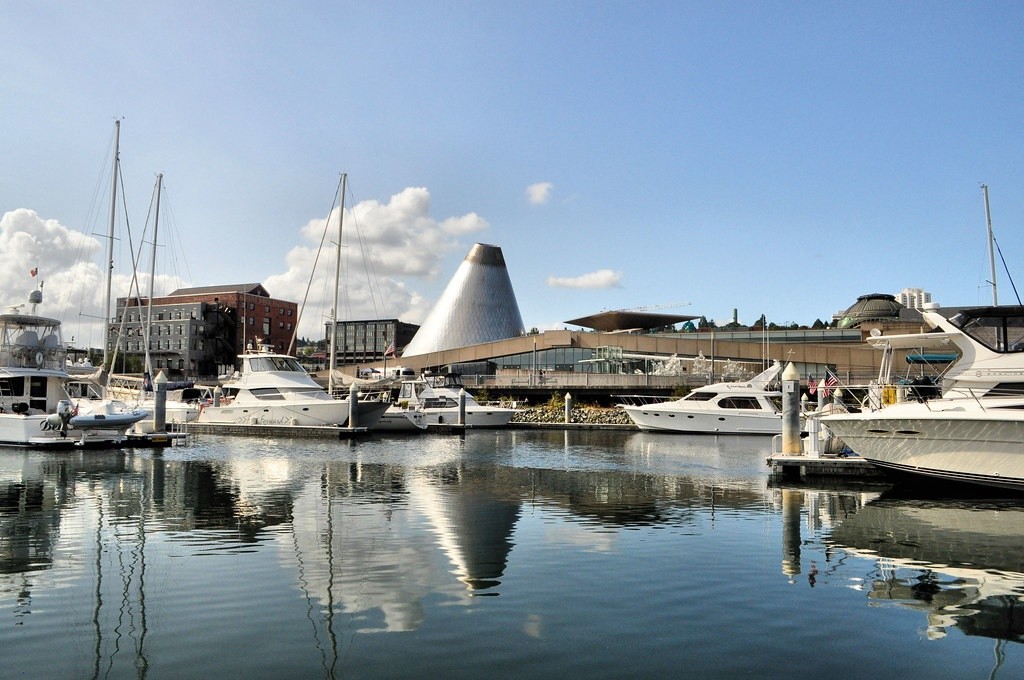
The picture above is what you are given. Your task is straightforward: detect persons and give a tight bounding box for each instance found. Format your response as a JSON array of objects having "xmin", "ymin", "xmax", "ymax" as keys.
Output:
[{"xmin": 538, "ymin": 370, "xmax": 547, "ymax": 385}]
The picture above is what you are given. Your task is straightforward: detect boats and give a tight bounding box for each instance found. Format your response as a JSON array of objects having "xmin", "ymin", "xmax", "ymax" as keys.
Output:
[
  {"xmin": 773, "ymin": 183, "xmax": 1024, "ymax": 498},
  {"xmin": 624, "ymin": 358, "xmax": 822, "ymax": 436},
  {"xmin": 0, "ymin": 114, "xmax": 521, "ymax": 449}
]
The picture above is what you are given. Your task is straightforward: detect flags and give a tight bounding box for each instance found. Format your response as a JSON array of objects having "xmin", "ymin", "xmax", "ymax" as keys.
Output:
[
  {"xmin": 384, "ymin": 342, "xmax": 395, "ymax": 355},
  {"xmin": 31, "ymin": 268, "xmax": 37, "ymax": 277},
  {"xmin": 807, "ymin": 374, "xmax": 817, "ymax": 394},
  {"xmin": 823, "ymin": 371, "xmax": 836, "ymax": 398}
]
